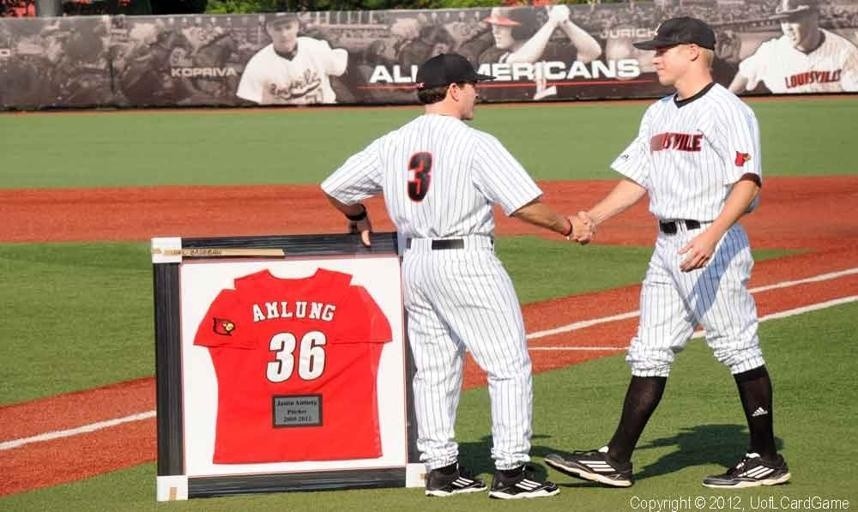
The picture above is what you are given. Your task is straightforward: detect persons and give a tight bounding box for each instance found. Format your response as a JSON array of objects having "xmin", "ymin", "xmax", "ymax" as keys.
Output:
[
  {"xmin": 476, "ymin": 8, "xmax": 603, "ymax": 78},
  {"xmin": 544, "ymin": 15, "xmax": 792, "ymax": 489},
  {"xmin": 233, "ymin": 13, "xmax": 378, "ymax": 107},
  {"xmin": 726, "ymin": 1, "xmax": 857, "ymax": 94},
  {"xmin": 319, "ymin": 51, "xmax": 597, "ymax": 500}
]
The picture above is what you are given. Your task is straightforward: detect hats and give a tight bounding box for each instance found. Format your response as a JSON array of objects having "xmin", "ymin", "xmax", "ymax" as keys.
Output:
[
  {"xmin": 483, "ymin": 8, "xmax": 535, "ymax": 26},
  {"xmin": 416, "ymin": 53, "xmax": 496, "ymax": 90},
  {"xmin": 769, "ymin": 0, "xmax": 819, "ymax": 19},
  {"xmin": 632, "ymin": 16, "xmax": 716, "ymax": 50},
  {"xmin": 264, "ymin": 13, "xmax": 297, "ymax": 25}
]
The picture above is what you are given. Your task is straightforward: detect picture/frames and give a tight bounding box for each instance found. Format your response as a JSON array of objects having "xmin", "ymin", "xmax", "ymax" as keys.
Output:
[{"xmin": 149, "ymin": 230, "xmax": 432, "ymax": 502}]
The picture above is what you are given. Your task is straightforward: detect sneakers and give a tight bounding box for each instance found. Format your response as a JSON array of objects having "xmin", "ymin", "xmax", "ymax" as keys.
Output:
[
  {"xmin": 544, "ymin": 446, "xmax": 635, "ymax": 488},
  {"xmin": 426, "ymin": 461, "xmax": 488, "ymax": 496},
  {"xmin": 488, "ymin": 464, "xmax": 561, "ymax": 499},
  {"xmin": 701, "ymin": 453, "xmax": 791, "ymax": 488}
]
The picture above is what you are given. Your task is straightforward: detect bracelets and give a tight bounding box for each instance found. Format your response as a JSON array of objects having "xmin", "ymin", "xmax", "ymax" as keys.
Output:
[
  {"xmin": 565, "ymin": 215, "xmax": 574, "ymax": 239},
  {"xmin": 344, "ymin": 206, "xmax": 369, "ymax": 224}
]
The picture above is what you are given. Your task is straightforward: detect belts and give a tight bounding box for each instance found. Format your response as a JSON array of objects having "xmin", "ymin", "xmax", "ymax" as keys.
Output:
[
  {"xmin": 659, "ymin": 221, "xmax": 700, "ymax": 234},
  {"xmin": 406, "ymin": 237, "xmax": 493, "ymax": 249}
]
